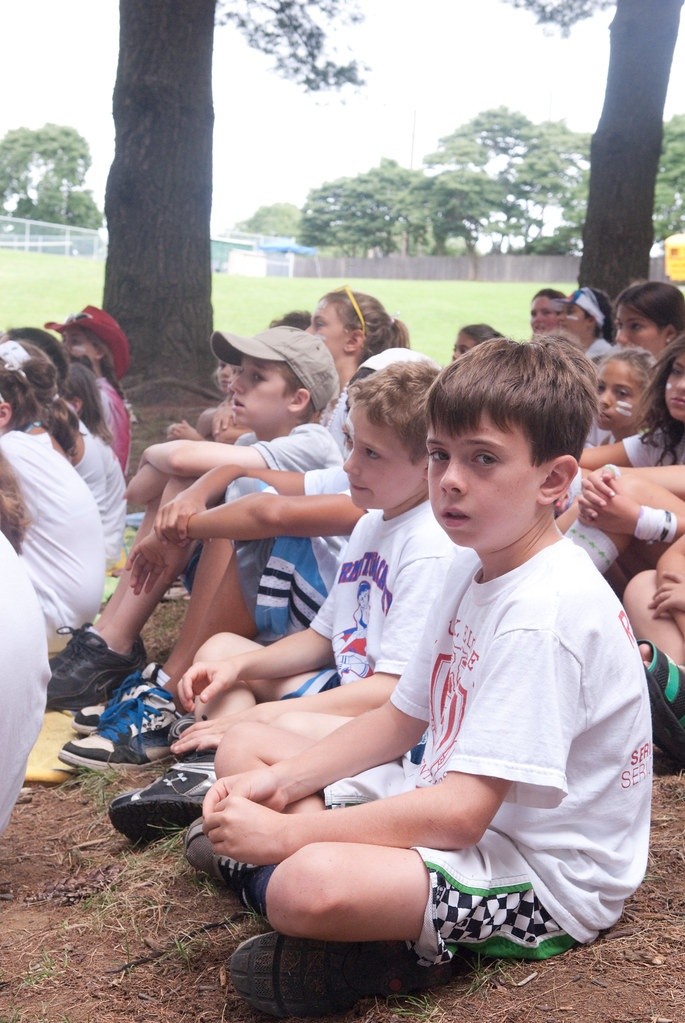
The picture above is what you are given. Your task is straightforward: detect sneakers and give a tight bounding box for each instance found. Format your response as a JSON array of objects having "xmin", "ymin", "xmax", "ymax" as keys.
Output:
[
  {"xmin": 71, "ymin": 662, "xmax": 171, "ymax": 734},
  {"xmin": 108, "ymin": 749, "xmax": 218, "ymax": 844},
  {"xmin": 227, "ymin": 931, "xmax": 455, "ymax": 1018},
  {"xmin": 184, "ymin": 816, "xmax": 280, "ymax": 919},
  {"xmin": 47, "ymin": 622, "xmax": 148, "ymax": 711},
  {"xmin": 58, "ymin": 686, "xmax": 195, "ymax": 773}
]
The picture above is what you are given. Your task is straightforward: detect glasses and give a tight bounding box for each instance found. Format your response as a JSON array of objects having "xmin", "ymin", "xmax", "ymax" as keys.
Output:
[
  {"xmin": 64, "ymin": 312, "xmax": 92, "ymax": 324},
  {"xmin": 334, "ymin": 284, "xmax": 366, "ymax": 334}
]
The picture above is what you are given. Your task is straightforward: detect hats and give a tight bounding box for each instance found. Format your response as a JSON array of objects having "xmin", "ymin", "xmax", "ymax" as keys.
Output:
[
  {"xmin": 357, "ymin": 347, "xmax": 443, "ymax": 372},
  {"xmin": 44, "ymin": 305, "xmax": 132, "ymax": 381},
  {"xmin": 210, "ymin": 325, "xmax": 340, "ymax": 412},
  {"xmin": 550, "ymin": 289, "xmax": 605, "ymax": 327}
]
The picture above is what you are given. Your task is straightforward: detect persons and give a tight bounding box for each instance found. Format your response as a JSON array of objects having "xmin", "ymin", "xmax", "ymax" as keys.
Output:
[
  {"xmin": 0, "ymin": 282, "xmax": 685, "ymax": 842},
  {"xmin": 183, "ymin": 332, "xmax": 653, "ymax": 1019}
]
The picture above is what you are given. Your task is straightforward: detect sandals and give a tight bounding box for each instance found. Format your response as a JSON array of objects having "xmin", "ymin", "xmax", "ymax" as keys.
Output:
[{"xmin": 637, "ymin": 638, "xmax": 685, "ymax": 763}]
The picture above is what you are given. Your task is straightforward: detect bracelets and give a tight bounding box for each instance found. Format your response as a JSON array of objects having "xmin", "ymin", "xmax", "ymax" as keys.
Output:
[
  {"xmin": 607, "ymin": 464, "xmax": 620, "ymax": 477},
  {"xmin": 634, "ymin": 506, "xmax": 678, "ymax": 544}
]
